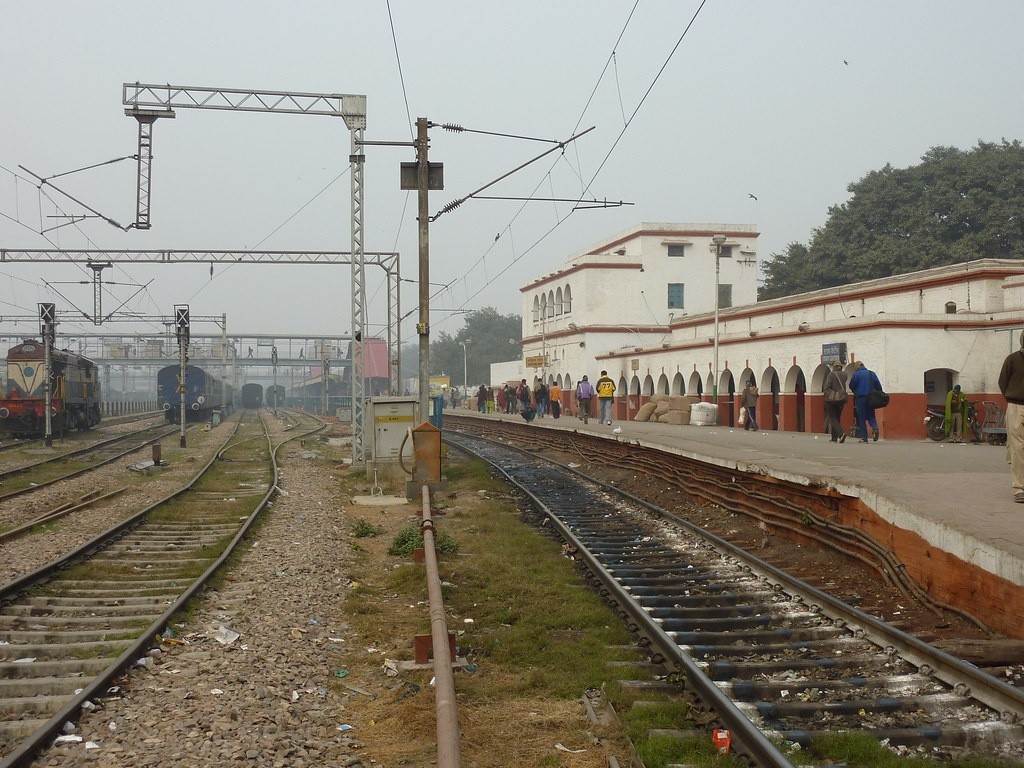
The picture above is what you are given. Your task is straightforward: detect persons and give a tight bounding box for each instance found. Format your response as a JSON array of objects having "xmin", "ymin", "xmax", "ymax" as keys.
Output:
[
  {"xmin": 405, "ymin": 389, "xmax": 409, "ymax": 396},
  {"xmin": 575, "ymin": 374, "xmax": 594, "ymax": 424},
  {"xmin": 849, "ymin": 361, "xmax": 882, "ymax": 443},
  {"xmin": 824, "ymin": 361, "xmax": 848, "ymax": 443},
  {"xmin": 596, "ymin": 371, "xmax": 616, "ymax": 425},
  {"xmin": 945, "ymin": 385, "xmax": 968, "ymax": 443},
  {"xmin": 392, "ymin": 389, "xmax": 396, "ymax": 395},
  {"xmin": 496, "ymin": 379, "xmax": 550, "ymax": 422},
  {"xmin": 742, "ymin": 380, "xmax": 759, "ymax": 431},
  {"xmin": 998, "ymin": 329, "xmax": 1024, "ymax": 503},
  {"xmin": 550, "ymin": 382, "xmax": 563, "ymax": 419},
  {"xmin": 443, "ymin": 388, "xmax": 460, "ymax": 409},
  {"xmin": 474, "ymin": 384, "xmax": 494, "ymax": 414}
]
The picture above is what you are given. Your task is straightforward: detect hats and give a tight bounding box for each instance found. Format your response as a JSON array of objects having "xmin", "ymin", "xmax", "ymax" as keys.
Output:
[
  {"xmin": 954, "ymin": 385, "xmax": 961, "ymax": 391},
  {"xmin": 855, "ymin": 361, "xmax": 865, "ymax": 370},
  {"xmin": 583, "ymin": 375, "xmax": 588, "ymax": 381},
  {"xmin": 833, "ymin": 361, "xmax": 843, "ymax": 367}
]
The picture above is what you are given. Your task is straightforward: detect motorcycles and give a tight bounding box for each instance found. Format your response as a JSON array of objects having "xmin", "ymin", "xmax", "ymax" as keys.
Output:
[{"xmin": 923, "ymin": 399, "xmax": 985, "ymax": 443}]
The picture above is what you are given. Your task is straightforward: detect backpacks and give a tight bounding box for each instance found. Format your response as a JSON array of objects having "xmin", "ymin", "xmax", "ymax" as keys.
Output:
[{"xmin": 521, "ymin": 385, "xmax": 530, "ymax": 401}]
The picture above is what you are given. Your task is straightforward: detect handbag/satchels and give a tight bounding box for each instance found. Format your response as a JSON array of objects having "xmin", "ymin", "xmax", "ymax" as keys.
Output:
[
  {"xmin": 867, "ymin": 391, "xmax": 889, "ymax": 409},
  {"xmin": 738, "ymin": 407, "xmax": 746, "ymax": 425},
  {"xmin": 824, "ymin": 389, "xmax": 844, "ymax": 401}
]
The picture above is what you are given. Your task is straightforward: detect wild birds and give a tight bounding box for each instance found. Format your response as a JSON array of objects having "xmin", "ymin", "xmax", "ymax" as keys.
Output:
[
  {"xmin": 614, "ymin": 426, "xmax": 622, "ymax": 435},
  {"xmin": 749, "ymin": 194, "xmax": 757, "ymax": 200},
  {"xmin": 844, "ymin": 60, "xmax": 848, "ymax": 65},
  {"xmin": 495, "ymin": 233, "xmax": 499, "ymax": 242}
]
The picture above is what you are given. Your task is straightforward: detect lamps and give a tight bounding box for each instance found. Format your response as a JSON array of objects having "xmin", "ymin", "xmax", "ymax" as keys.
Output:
[
  {"xmin": 568, "ymin": 322, "xmax": 583, "ymax": 334},
  {"xmin": 509, "ymin": 339, "xmax": 524, "ymax": 347}
]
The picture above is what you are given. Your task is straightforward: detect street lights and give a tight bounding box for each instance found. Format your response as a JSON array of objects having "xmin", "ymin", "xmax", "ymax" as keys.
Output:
[
  {"xmin": 458, "ymin": 341, "xmax": 467, "ymax": 390},
  {"xmin": 712, "ymin": 234, "xmax": 726, "ymax": 404}
]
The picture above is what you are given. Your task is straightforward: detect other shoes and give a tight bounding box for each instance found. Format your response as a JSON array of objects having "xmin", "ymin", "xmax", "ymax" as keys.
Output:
[
  {"xmin": 597, "ymin": 420, "xmax": 603, "ymax": 425},
  {"xmin": 839, "ymin": 433, "xmax": 846, "ymax": 443},
  {"xmin": 1013, "ymin": 493, "xmax": 1024, "ymax": 503},
  {"xmin": 830, "ymin": 439, "xmax": 837, "ymax": 444},
  {"xmin": 752, "ymin": 428, "xmax": 758, "ymax": 431},
  {"xmin": 872, "ymin": 429, "xmax": 879, "ymax": 441},
  {"xmin": 858, "ymin": 438, "xmax": 868, "ymax": 444},
  {"xmin": 607, "ymin": 419, "xmax": 612, "ymax": 425},
  {"xmin": 743, "ymin": 429, "xmax": 749, "ymax": 431}
]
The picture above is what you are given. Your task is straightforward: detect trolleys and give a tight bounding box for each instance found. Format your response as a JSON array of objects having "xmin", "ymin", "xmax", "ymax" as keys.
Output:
[{"xmin": 982, "ymin": 408, "xmax": 1007, "ymax": 445}]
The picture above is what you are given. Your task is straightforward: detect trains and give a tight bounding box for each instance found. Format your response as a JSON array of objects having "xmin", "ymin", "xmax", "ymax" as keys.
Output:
[
  {"xmin": 157, "ymin": 364, "xmax": 239, "ymax": 422},
  {"xmin": 265, "ymin": 385, "xmax": 285, "ymax": 406},
  {"xmin": 0, "ymin": 338, "xmax": 102, "ymax": 438},
  {"xmin": 241, "ymin": 383, "xmax": 263, "ymax": 407}
]
